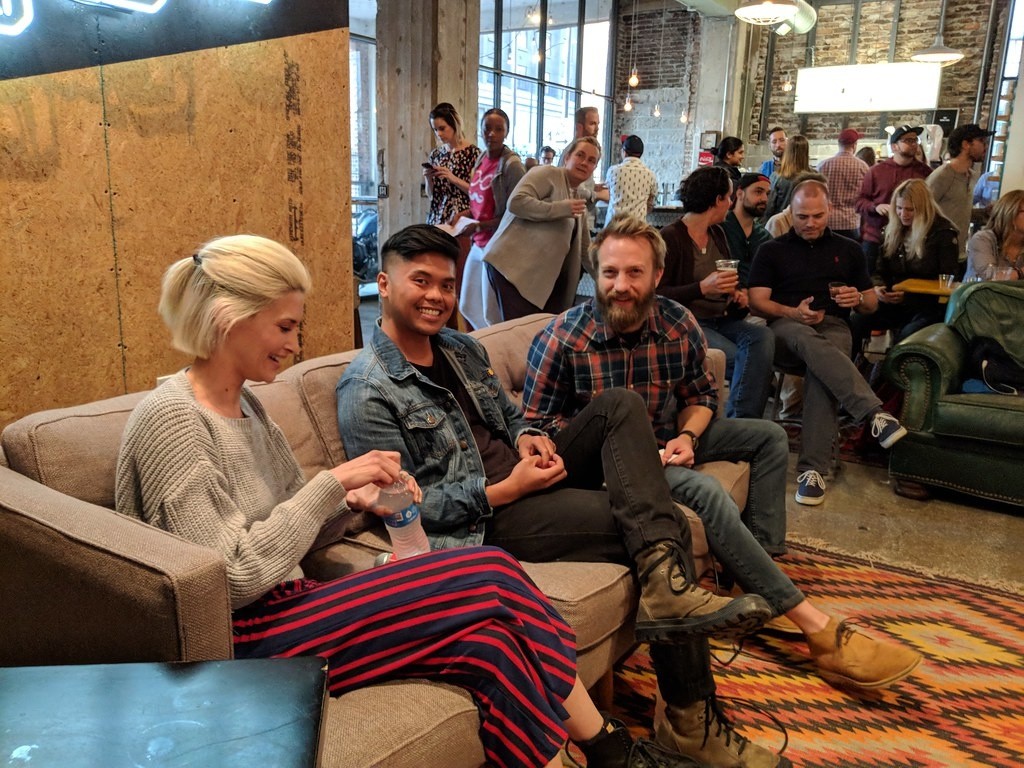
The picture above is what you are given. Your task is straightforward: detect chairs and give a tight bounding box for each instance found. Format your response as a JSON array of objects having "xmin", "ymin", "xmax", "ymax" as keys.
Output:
[{"xmin": 773, "ymin": 321, "xmax": 862, "ymax": 469}]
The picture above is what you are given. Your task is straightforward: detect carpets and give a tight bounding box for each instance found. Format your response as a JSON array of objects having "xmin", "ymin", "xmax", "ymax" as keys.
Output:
[{"xmin": 556, "ymin": 531, "xmax": 1024, "ymax": 768}]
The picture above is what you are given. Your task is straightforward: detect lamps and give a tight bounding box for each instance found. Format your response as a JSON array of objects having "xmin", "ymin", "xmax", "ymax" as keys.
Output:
[
  {"xmin": 772, "ymin": 22, "xmax": 792, "ymax": 36},
  {"xmin": 911, "ymin": 0, "xmax": 965, "ymax": 67},
  {"xmin": 735, "ymin": 0, "xmax": 799, "ymax": 25}
]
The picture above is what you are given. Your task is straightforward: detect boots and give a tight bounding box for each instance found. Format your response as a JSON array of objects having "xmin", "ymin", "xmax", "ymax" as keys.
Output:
[
  {"xmin": 564, "ymin": 715, "xmax": 700, "ymax": 768},
  {"xmin": 654, "ymin": 696, "xmax": 793, "ymax": 768},
  {"xmin": 632, "ymin": 540, "xmax": 772, "ymax": 644}
]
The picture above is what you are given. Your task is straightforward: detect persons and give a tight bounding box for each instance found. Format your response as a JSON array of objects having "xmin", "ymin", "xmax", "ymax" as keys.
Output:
[
  {"xmin": 655, "ymin": 166, "xmax": 802, "ymax": 438},
  {"xmin": 961, "ymin": 190, "xmax": 1024, "ymax": 284},
  {"xmin": 816, "ymin": 128, "xmax": 875, "ymax": 240},
  {"xmin": 423, "ymin": 103, "xmax": 524, "ymax": 331},
  {"xmin": 756, "ymin": 126, "xmax": 829, "ymax": 237},
  {"xmin": 522, "ymin": 145, "xmax": 555, "ymax": 172},
  {"xmin": 712, "ymin": 135, "xmax": 745, "ymax": 208},
  {"xmin": 854, "ymin": 124, "xmax": 933, "ymax": 266},
  {"xmin": 520, "ymin": 217, "xmax": 923, "ymax": 692},
  {"xmin": 334, "ymin": 223, "xmax": 793, "ymax": 768},
  {"xmin": 882, "ymin": 178, "xmax": 960, "ymax": 348},
  {"xmin": 923, "ymin": 124, "xmax": 997, "ymax": 283},
  {"xmin": 748, "ymin": 182, "xmax": 908, "ymax": 506},
  {"xmin": 555, "ymin": 106, "xmax": 610, "ymax": 307},
  {"xmin": 604, "ymin": 134, "xmax": 658, "ymax": 226},
  {"xmin": 482, "ymin": 138, "xmax": 603, "ymax": 323},
  {"xmin": 113, "ymin": 233, "xmax": 707, "ymax": 768},
  {"xmin": 973, "ymin": 168, "xmax": 1001, "ymax": 209}
]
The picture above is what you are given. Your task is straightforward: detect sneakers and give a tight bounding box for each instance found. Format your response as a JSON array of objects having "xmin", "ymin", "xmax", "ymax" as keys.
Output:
[
  {"xmin": 794, "ymin": 469, "xmax": 826, "ymax": 506},
  {"xmin": 869, "ymin": 409, "xmax": 907, "ymax": 449},
  {"xmin": 805, "ymin": 614, "xmax": 922, "ymax": 690},
  {"xmin": 762, "ymin": 611, "xmax": 804, "ymax": 634}
]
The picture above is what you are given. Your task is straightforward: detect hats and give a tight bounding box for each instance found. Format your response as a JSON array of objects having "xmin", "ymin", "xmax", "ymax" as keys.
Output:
[
  {"xmin": 838, "ymin": 128, "xmax": 865, "ymax": 145},
  {"xmin": 727, "ymin": 173, "xmax": 771, "ymax": 210},
  {"xmin": 620, "ymin": 134, "xmax": 644, "ymax": 157},
  {"xmin": 891, "ymin": 125, "xmax": 923, "ymax": 143},
  {"xmin": 947, "ymin": 124, "xmax": 996, "ymax": 154}
]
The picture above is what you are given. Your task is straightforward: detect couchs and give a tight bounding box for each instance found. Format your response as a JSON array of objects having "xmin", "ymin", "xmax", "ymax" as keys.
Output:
[{"xmin": 885, "ymin": 279, "xmax": 1024, "ymax": 507}]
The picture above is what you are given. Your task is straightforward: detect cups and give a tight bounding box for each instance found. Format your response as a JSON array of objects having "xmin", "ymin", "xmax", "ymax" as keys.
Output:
[
  {"xmin": 568, "ymin": 187, "xmax": 588, "ymax": 218},
  {"xmin": 715, "ymin": 259, "xmax": 739, "ymax": 292},
  {"xmin": 828, "ymin": 282, "xmax": 847, "ymax": 300},
  {"xmin": 965, "ymin": 277, "xmax": 982, "ymax": 283},
  {"xmin": 939, "ymin": 275, "xmax": 955, "ymax": 289},
  {"xmin": 991, "ymin": 266, "xmax": 1013, "ymax": 281}
]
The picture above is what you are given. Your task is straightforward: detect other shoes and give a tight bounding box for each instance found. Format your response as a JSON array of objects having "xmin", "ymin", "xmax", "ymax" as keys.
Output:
[{"xmin": 768, "ymin": 384, "xmax": 784, "ymax": 405}]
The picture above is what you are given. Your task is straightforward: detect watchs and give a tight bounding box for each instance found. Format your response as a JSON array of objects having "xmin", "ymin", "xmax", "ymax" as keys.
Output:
[
  {"xmin": 678, "ymin": 430, "xmax": 699, "ymax": 450},
  {"xmin": 859, "ymin": 293, "xmax": 864, "ymax": 305}
]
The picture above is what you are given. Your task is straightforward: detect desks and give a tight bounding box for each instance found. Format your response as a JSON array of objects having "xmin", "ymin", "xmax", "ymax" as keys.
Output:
[
  {"xmin": 892, "ymin": 278, "xmax": 961, "ymax": 296},
  {"xmin": 0, "ymin": 655, "xmax": 330, "ymax": 768}
]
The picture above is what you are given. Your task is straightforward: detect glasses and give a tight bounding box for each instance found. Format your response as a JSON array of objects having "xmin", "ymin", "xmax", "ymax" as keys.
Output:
[
  {"xmin": 973, "ymin": 136, "xmax": 987, "ymax": 143},
  {"xmin": 572, "ymin": 149, "xmax": 598, "ymax": 167},
  {"xmin": 898, "ymin": 137, "xmax": 918, "ymax": 145},
  {"xmin": 539, "ymin": 155, "xmax": 553, "ymax": 162}
]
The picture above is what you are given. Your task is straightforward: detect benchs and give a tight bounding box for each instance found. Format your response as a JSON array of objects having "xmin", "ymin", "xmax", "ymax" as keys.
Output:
[{"xmin": 0, "ymin": 313, "xmax": 751, "ymax": 768}]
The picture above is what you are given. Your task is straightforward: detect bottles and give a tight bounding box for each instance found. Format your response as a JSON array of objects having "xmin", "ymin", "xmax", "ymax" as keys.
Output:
[{"xmin": 378, "ymin": 479, "xmax": 431, "ymax": 561}]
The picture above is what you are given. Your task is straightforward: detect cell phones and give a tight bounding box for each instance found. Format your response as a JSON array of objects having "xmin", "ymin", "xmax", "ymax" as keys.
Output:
[{"xmin": 421, "ymin": 163, "xmax": 435, "ymax": 174}]
